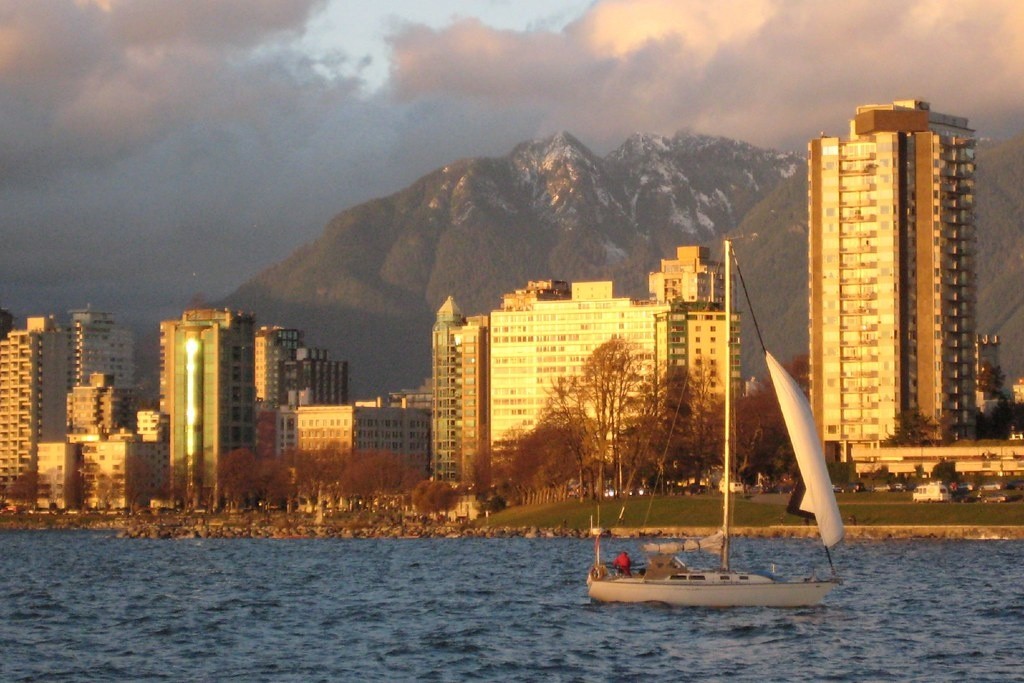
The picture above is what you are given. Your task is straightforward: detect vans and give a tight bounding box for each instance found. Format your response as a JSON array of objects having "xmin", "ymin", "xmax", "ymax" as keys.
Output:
[
  {"xmin": 729, "ymin": 481, "xmax": 744, "ymax": 493},
  {"xmin": 912, "ymin": 482, "xmax": 949, "ymax": 503}
]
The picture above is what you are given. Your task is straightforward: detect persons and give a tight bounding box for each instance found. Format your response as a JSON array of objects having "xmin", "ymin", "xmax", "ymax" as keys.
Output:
[
  {"xmin": 412, "ymin": 511, "xmax": 451, "ymax": 527},
  {"xmin": 612, "ymin": 551, "xmax": 632, "ymax": 578}
]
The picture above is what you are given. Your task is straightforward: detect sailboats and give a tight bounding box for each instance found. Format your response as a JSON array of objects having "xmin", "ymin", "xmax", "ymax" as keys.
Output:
[{"xmin": 588, "ymin": 238, "xmax": 845, "ymax": 608}]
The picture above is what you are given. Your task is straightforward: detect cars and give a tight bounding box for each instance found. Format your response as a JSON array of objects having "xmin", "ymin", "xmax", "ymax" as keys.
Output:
[
  {"xmin": 831, "ymin": 482, "xmax": 843, "ymax": 492},
  {"xmin": 981, "ymin": 493, "xmax": 1009, "ymax": 503}
]
